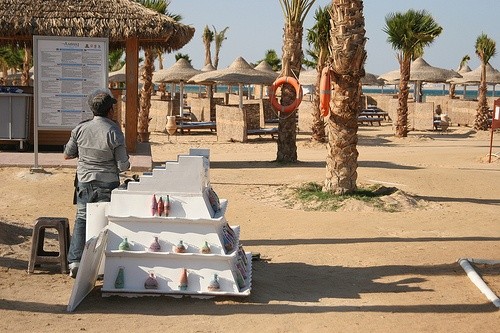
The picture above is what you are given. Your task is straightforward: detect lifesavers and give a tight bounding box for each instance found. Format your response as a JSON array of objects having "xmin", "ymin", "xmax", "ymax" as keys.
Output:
[
  {"xmin": 269, "ymin": 76, "xmax": 303, "ymax": 114},
  {"xmin": 319, "ymin": 66, "xmax": 331, "ymax": 116}
]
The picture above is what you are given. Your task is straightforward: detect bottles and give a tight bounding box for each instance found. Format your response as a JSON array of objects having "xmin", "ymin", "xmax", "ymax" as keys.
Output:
[
  {"xmin": 208, "ymin": 273, "xmax": 220, "ymax": 291},
  {"xmin": 119, "ymin": 238, "xmax": 129, "ymax": 250},
  {"xmin": 115, "ymin": 267, "xmax": 124, "ymax": 289},
  {"xmin": 145, "ymin": 272, "xmax": 159, "ymax": 289},
  {"xmin": 202, "ymin": 241, "xmax": 210, "ymax": 254},
  {"xmin": 236, "ymin": 244, "xmax": 248, "ymax": 289},
  {"xmin": 222, "ymin": 221, "xmax": 236, "ymax": 251},
  {"xmin": 179, "ymin": 268, "xmax": 188, "ymax": 290},
  {"xmin": 148, "ymin": 236, "xmax": 161, "ymax": 252},
  {"xmin": 175, "ymin": 240, "xmax": 186, "ymax": 253}
]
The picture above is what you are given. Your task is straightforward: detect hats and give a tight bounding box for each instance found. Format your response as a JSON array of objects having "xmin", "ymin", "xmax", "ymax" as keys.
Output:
[{"xmin": 89, "ymin": 93, "xmax": 117, "ymax": 115}]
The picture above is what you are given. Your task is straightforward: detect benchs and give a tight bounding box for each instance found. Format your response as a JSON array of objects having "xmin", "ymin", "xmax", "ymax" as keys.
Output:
[
  {"xmin": 358, "ymin": 106, "xmax": 389, "ymax": 127},
  {"xmin": 247, "ymin": 128, "xmax": 279, "ymax": 141},
  {"xmin": 175, "ymin": 115, "xmax": 216, "ymax": 134}
]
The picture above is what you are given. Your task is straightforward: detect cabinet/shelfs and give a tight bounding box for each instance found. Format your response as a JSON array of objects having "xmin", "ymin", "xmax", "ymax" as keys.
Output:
[{"xmin": 100, "ymin": 148, "xmax": 253, "ymax": 299}]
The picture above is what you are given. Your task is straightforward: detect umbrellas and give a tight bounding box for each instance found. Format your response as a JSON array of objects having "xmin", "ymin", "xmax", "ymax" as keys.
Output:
[
  {"xmin": 107, "ymin": 56, "xmax": 280, "ymax": 118},
  {"xmin": 299, "ymin": 67, "xmax": 385, "ymax": 87},
  {"xmin": 446, "ymin": 62, "xmax": 500, "ymax": 98},
  {"xmin": 378, "ymin": 58, "xmax": 463, "ymax": 101}
]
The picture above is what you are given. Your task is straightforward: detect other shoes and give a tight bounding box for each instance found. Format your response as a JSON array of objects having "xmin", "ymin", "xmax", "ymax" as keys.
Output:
[{"xmin": 68, "ymin": 261, "xmax": 81, "ymax": 277}]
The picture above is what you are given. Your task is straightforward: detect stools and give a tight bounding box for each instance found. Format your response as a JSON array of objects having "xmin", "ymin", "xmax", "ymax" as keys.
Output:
[{"xmin": 28, "ymin": 217, "xmax": 71, "ymax": 274}]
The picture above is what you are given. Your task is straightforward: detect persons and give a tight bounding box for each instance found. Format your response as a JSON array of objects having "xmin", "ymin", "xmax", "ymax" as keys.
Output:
[{"xmin": 64, "ymin": 93, "xmax": 130, "ymax": 278}]
